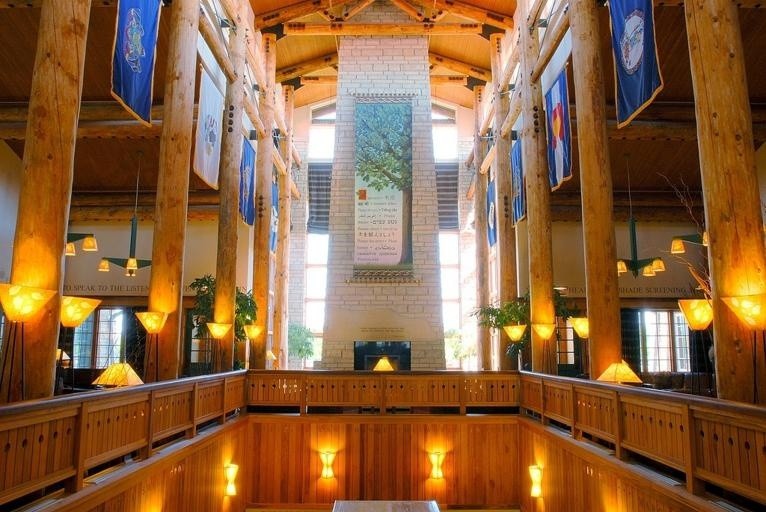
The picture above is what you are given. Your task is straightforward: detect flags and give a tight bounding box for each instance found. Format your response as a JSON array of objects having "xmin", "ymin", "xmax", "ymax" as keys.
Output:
[
  {"xmin": 509, "ymin": 138, "xmax": 527, "ymax": 228},
  {"xmin": 191, "ymin": 61, "xmax": 228, "ymax": 192},
  {"xmin": 603, "ymin": 1, "xmax": 665, "ymax": 130},
  {"xmin": 485, "ymin": 176, "xmax": 498, "ymax": 248},
  {"xmin": 109, "ymin": 0, "xmax": 165, "ymax": 129},
  {"xmin": 238, "ymin": 134, "xmax": 257, "ymax": 227},
  {"xmin": 544, "ymin": 65, "xmax": 574, "ymax": 192},
  {"xmin": 269, "ymin": 181, "xmax": 279, "ymax": 256}
]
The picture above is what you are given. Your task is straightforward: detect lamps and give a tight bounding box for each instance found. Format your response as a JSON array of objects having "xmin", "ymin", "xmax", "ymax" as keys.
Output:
[
  {"xmin": 530, "ymin": 323, "xmax": 558, "ymax": 342},
  {"xmin": 56, "ymin": 350, "xmax": 70, "ymax": 361},
  {"xmin": 125, "ymin": 267, "xmax": 138, "ymax": 276},
  {"xmin": 317, "ymin": 444, "xmax": 339, "ymax": 483},
  {"xmin": 502, "ymin": 324, "xmax": 527, "ymax": 342},
  {"xmin": 82, "ymin": 235, "xmax": 98, "ymax": 253},
  {"xmin": 427, "ymin": 447, "xmax": 449, "ymax": 485},
  {"xmin": 701, "ymin": 231, "xmax": 707, "ymax": 246},
  {"xmin": 373, "ymin": 357, "xmax": 395, "ymax": 372},
  {"xmin": 529, "ymin": 462, "xmax": 542, "ymax": 501},
  {"xmin": 678, "ymin": 296, "xmax": 716, "ymax": 330},
  {"xmin": 67, "ymin": 244, "xmax": 77, "ymax": 257},
  {"xmin": 597, "ymin": 360, "xmax": 642, "ymax": 385},
  {"xmin": 2, "ymin": 283, "xmax": 57, "ymax": 324},
  {"xmin": 243, "ymin": 323, "xmax": 265, "ymax": 339},
  {"xmin": 617, "ymin": 260, "xmax": 626, "ymax": 273},
  {"xmin": 652, "ymin": 259, "xmax": 665, "ymax": 271},
  {"xmin": 669, "ymin": 239, "xmax": 685, "ymax": 255},
  {"xmin": 567, "ymin": 314, "xmax": 589, "ymax": 340},
  {"xmin": 204, "ymin": 322, "xmax": 233, "ymax": 339},
  {"xmin": 223, "ymin": 459, "xmax": 239, "ymax": 496},
  {"xmin": 98, "ymin": 258, "xmax": 111, "ymax": 274},
  {"xmin": 642, "ymin": 266, "xmax": 656, "ymax": 276},
  {"xmin": 718, "ymin": 291, "xmax": 766, "ymax": 336},
  {"xmin": 61, "ymin": 296, "xmax": 103, "ymax": 331},
  {"xmin": 92, "ymin": 362, "xmax": 144, "ymax": 387},
  {"xmin": 128, "ymin": 259, "xmax": 141, "ymax": 270},
  {"xmin": 136, "ymin": 311, "xmax": 170, "ymax": 335}
]
